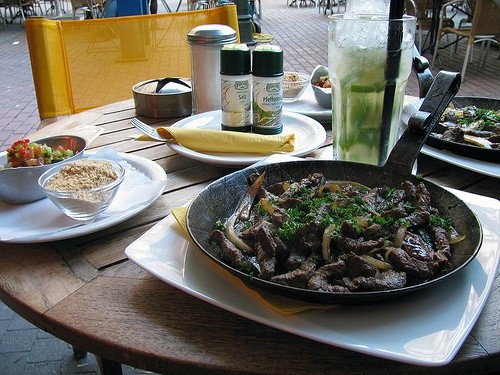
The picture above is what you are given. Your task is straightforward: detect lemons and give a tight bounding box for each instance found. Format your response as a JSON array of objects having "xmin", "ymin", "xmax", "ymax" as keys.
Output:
[{"xmin": 328, "ymin": 68, "xmax": 388, "ymax": 165}]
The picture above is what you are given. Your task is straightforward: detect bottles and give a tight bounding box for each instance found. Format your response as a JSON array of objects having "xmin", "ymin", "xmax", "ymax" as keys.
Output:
[
  {"xmin": 253, "ymin": 45, "xmax": 283, "ymax": 134},
  {"xmin": 219, "ymin": 44, "xmax": 253, "ymax": 133},
  {"xmin": 186, "ymin": 24, "xmax": 239, "ymax": 117}
]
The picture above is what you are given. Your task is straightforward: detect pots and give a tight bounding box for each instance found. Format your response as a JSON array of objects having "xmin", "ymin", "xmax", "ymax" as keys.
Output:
[
  {"xmin": 186, "ymin": 69, "xmax": 483, "ymax": 306},
  {"xmin": 400, "ymin": 43, "xmax": 500, "ymax": 160}
]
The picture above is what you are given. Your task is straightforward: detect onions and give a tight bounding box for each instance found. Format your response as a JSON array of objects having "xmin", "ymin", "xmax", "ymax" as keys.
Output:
[
  {"xmin": 224, "ymin": 172, "xmax": 408, "ymax": 268},
  {"xmin": 441, "ymin": 103, "xmax": 493, "ymax": 150}
]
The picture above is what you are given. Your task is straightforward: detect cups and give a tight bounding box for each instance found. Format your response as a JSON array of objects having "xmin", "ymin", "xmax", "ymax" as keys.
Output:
[{"xmin": 327, "ymin": 14, "xmax": 416, "ymax": 166}]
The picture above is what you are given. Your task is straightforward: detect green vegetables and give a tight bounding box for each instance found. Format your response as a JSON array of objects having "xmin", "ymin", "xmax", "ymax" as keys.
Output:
[
  {"xmin": 213, "ymin": 179, "xmax": 455, "ymax": 241},
  {"xmin": 455, "ymin": 106, "xmax": 500, "ymax": 132},
  {"xmin": 237, "ymin": 257, "xmax": 257, "ymax": 283}
]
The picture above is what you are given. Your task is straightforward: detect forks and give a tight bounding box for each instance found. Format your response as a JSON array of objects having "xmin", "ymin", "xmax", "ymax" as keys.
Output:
[{"xmin": 130, "ymin": 117, "xmax": 174, "ymax": 142}]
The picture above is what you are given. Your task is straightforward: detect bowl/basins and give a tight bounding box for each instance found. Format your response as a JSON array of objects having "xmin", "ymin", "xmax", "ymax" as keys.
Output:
[
  {"xmin": 281, "ymin": 71, "xmax": 310, "ymax": 104},
  {"xmin": 309, "ymin": 65, "xmax": 332, "ymax": 109},
  {"xmin": 38, "ymin": 158, "xmax": 125, "ymax": 221}
]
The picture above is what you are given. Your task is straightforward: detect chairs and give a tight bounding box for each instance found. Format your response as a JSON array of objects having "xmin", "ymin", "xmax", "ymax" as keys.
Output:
[{"xmin": 0, "ymin": 0, "xmax": 500, "ymax": 119}]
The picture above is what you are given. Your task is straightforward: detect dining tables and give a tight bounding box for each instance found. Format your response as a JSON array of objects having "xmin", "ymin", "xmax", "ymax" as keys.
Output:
[{"xmin": 0, "ymin": 76, "xmax": 500, "ymax": 375}]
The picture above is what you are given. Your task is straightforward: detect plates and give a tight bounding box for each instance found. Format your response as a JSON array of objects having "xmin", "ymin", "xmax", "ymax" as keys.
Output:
[
  {"xmin": 125, "ymin": 152, "xmax": 500, "ymax": 366},
  {"xmin": 166, "ymin": 108, "xmax": 326, "ymax": 167},
  {"xmin": 0, "ymin": 124, "xmax": 105, "ymax": 205},
  {"xmin": 0, "ymin": 149, "xmax": 168, "ymax": 242},
  {"xmin": 416, "ymin": 138, "xmax": 500, "ymax": 179}
]
